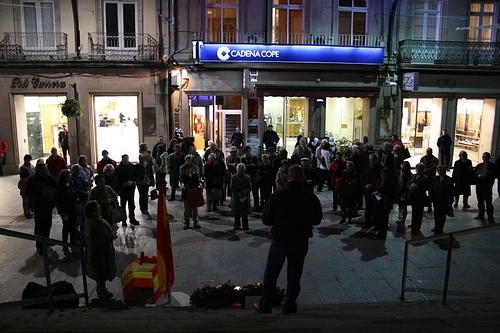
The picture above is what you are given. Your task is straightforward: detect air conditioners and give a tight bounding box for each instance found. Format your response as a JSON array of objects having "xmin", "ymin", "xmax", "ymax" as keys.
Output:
[
  {"xmin": 245, "ymin": 32, "xmax": 264, "ymax": 44},
  {"xmin": 311, "ymin": 35, "xmax": 334, "ymax": 45}
]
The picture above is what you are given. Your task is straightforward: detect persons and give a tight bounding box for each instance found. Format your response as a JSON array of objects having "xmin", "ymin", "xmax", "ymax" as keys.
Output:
[
  {"xmin": 59, "ymin": 125, "xmax": 70, "ymax": 163},
  {"xmin": 474, "ymin": 152, "xmax": 497, "ymax": 223},
  {"xmin": 119, "ymin": 113, "xmax": 124, "ymax": 123},
  {"xmin": 230, "ymin": 163, "xmax": 252, "ymax": 230},
  {"xmin": 230, "ymin": 128, "xmax": 244, "ymax": 149},
  {"xmin": 18, "ymin": 143, "xmax": 155, "ymax": 254},
  {"xmin": 253, "ymin": 163, "xmax": 323, "ymax": 313},
  {"xmin": 0, "ymin": 137, "xmax": 8, "ymax": 176},
  {"xmin": 437, "ymin": 129, "xmax": 452, "ymax": 171},
  {"xmin": 427, "ymin": 166, "xmax": 454, "ymax": 235},
  {"xmin": 152, "ymin": 135, "xmax": 321, "ymax": 229},
  {"xmin": 263, "ymin": 125, "xmax": 279, "ymax": 151},
  {"xmin": 99, "ymin": 117, "xmax": 108, "ymax": 127},
  {"xmin": 292, "ymin": 130, "xmax": 500, "ymax": 237},
  {"xmin": 84, "ymin": 200, "xmax": 117, "ymax": 299}
]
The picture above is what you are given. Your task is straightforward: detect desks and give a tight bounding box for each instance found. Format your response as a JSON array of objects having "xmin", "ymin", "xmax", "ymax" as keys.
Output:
[{"xmin": 121, "ymin": 256, "xmax": 167, "ymax": 307}]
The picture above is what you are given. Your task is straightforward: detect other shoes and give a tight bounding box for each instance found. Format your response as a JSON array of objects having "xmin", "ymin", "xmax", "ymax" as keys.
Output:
[
  {"xmin": 253, "ymin": 303, "xmax": 273, "ymax": 314},
  {"xmin": 206, "ymin": 209, "xmax": 211, "ymax": 212},
  {"xmin": 282, "ymin": 304, "xmax": 298, "ymax": 316},
  {"xmin": 183, "ymin": 225, "xmax": 189, "ymax": 230},
  {"xmin": 122, "ymin": 220, "xmax": 127, "ymax": 226},
  {"xmin": 168, "ymin": 197, "xmax": 175, "ymax": 201},
  {"xmin": 142, "ymin": 210, "xmax": 149, "ymax": 214},
  {"xmin": 129, "ymin": 218, "xmax": 139, "ymax": 225},
  {"xmin": 96, "ymin": 288, "xmax": 114, "ymax": 299},
  {"xmin": 194, "ymin": 224, "xmax": 201, "ymax": 228}
]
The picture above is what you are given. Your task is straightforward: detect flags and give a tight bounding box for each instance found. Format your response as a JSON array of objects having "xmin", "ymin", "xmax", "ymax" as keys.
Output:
[{"xmin": 157, "ymin": 184, "xmax": 175, "ymax": 293}]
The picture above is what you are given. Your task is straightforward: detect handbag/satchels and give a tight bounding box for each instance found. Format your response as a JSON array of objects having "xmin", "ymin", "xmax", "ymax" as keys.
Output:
[
  {"xmin": 150, "ymin": 185, "xmax": 158, "ymax": 200},
  {"xmin": 187, "ymin": 186, "xmax": 205, "ymax": 208},
  {"xmin": 110, "ymin": 197, "xmax": 123, "ymax": 223}
]
